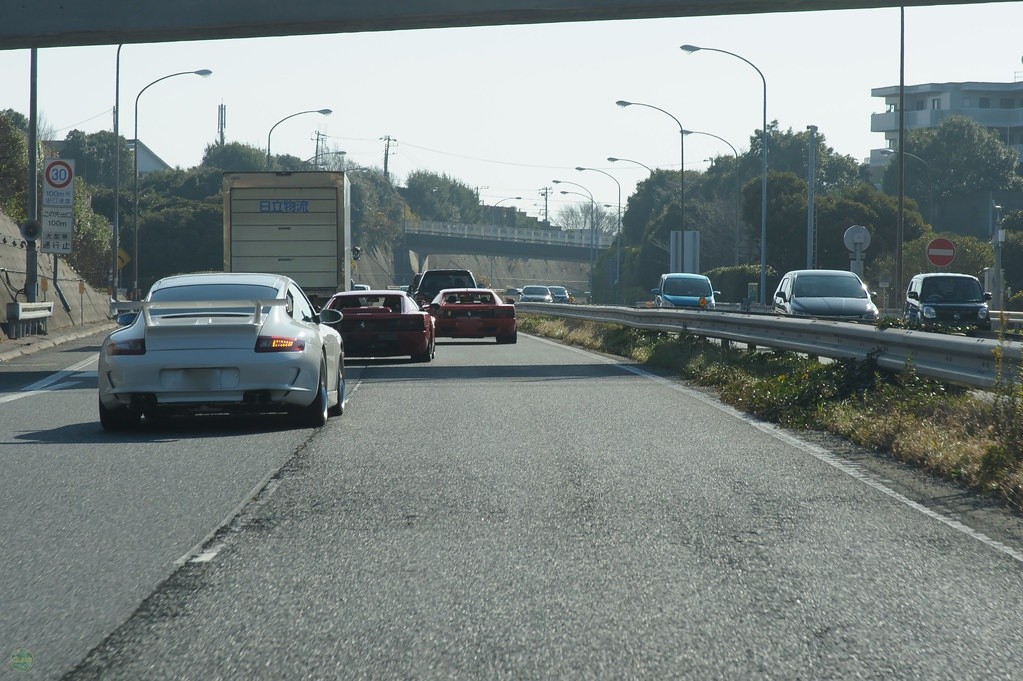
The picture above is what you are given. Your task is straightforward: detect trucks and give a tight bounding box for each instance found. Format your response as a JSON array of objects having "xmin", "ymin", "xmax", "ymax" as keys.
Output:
[{"xmin": 220, "ymin": 170, "xmax": 361, "ymax": 312}]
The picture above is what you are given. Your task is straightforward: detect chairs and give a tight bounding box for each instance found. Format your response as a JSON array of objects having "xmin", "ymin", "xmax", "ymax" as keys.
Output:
[
  {"xmin": 460, "ymin": 296, "xmax": 474, "ymax": 302},
  {"xmin": 341, "ymin": 297, "xmax": 361, "ymax": 307},
  {"xmin": 382, "ymin": 296, "xmax": 401, "ymax": 312}
]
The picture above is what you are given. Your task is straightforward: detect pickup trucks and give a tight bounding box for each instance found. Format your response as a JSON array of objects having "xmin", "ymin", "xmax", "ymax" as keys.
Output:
[{"xmin": 406, "ymin": 268, "xmax": 486, "ymax": 310}]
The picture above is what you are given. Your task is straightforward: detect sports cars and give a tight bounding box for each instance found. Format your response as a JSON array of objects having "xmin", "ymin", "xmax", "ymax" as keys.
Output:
[
  {"xmin": 424, "ymin": 286, "xmax": 518, "ymax": 345},
  {"xmin": 314, "ymin": 288, "xmax": 440, "ymax": 363},
  {"xmin": 96, "ymin": 271, "xmax": 346, "ymax": 430}
]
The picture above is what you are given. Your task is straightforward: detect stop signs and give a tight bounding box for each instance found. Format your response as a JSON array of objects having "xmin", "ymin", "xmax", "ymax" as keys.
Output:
[{"xmin": 926, "ymin": 238, "xmax": 956, "ymax": 268}]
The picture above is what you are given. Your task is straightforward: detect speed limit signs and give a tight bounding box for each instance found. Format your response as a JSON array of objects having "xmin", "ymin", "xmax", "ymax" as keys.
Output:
[{"xmin": 43, "ymin": 158, "xmax": 75, "ymax": 206}]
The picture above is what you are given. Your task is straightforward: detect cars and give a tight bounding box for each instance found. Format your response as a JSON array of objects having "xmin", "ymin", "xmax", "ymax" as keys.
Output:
[
  {"xmin": 546, "ymin": 285, "xmax": 570, "ymax": 305},
  {"xmin": 519, "ymin": 284, "xmax": 554, "ymax": 304},
  {"xmin": 771, "ymin": 268, "xmax": 880, "ymax": 325},
  {"xmin": 506, "ymin": 288, "xmax": 523, "ymax": 295},
  {"xmin": 649, "ymin": 271, "xmax": 716, "ymax": 311}
]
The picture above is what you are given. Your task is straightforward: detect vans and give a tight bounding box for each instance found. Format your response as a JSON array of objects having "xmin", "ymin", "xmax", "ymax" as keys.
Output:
[{"xmin": 903, "ymin": 272, "xmax": 993, "ymax": 337}]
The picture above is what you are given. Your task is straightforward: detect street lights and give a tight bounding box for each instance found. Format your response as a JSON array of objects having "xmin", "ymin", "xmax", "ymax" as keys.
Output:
[
  {"xmin": 552, "ymin": 179, "xmax": 594, "ymax": 305},
  {"xmin": 559, "ymin": 191, "xmax": 598, "ymax": 305},
  {"xmin": 608, "ymin": 157, "xmax": 657, "ymax": 206},
  {"xmin": 615, "ymin": 100, "xmax": 685, "ymax": 273},
  {"xmin": 575, "ymin": 167, "xmax": 621, "ymax": 306},
  {"xmin": 679, "ymin": 129, "xmax": 740, "ymax": 303},
  {"xmin": 879, "ymin": 148, "xmax": 934, "ymax": 238},
  {"xmin": 679, "ymin": 44, "xmax": 768, "ymax": 305},
  {"xmin": 491, "ymin": 197, "xmax": 522, "ymax": 226},
  {"xmin": 132, "ymin": 68, "xmax": 212, "ymax": 301},
  {"xmin": 268, "ymin": 108, "xmax": 333, "ymax": 173}
]
